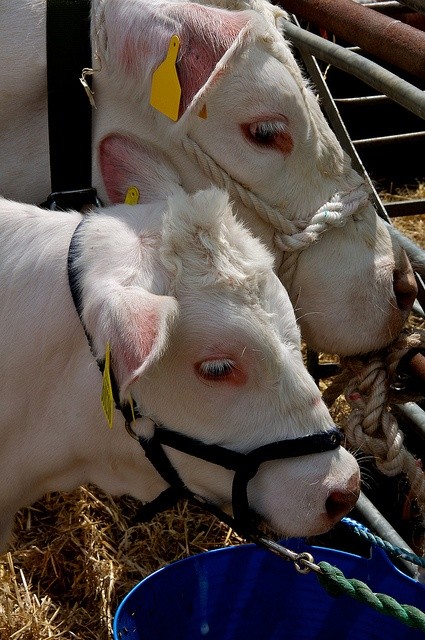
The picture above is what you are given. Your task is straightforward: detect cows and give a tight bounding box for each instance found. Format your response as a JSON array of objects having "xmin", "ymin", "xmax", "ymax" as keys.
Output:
[
  {"xmin": 0, "ymin": 189, "xmax": 378, "ymax": 555},
  {"xmin": 0, "ymin": 0, "xmax": 419, "ymax": 357}
]
